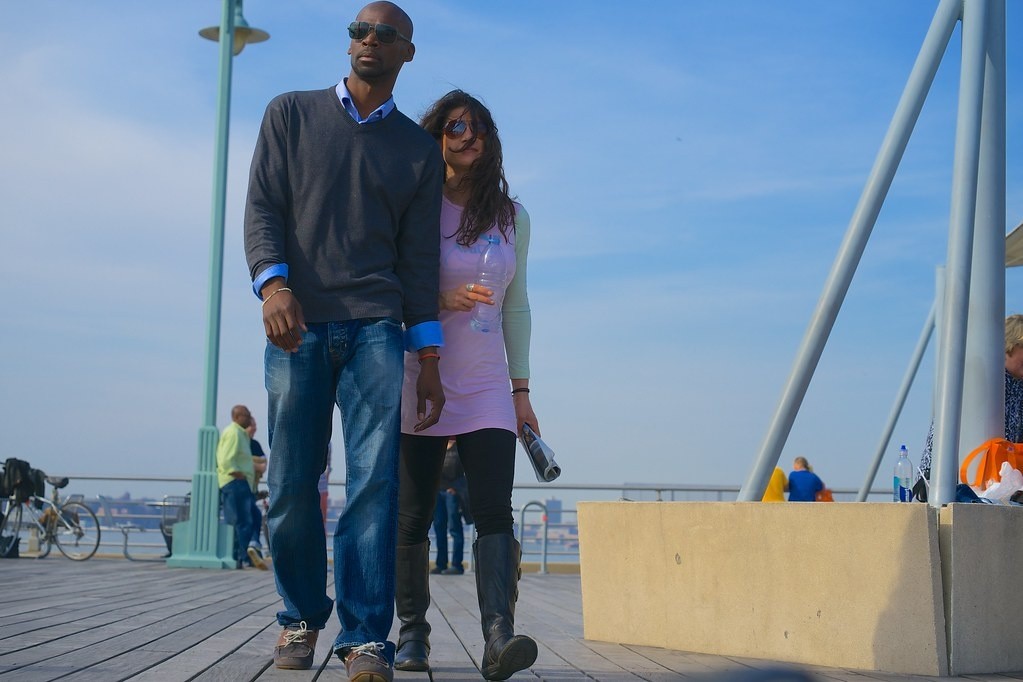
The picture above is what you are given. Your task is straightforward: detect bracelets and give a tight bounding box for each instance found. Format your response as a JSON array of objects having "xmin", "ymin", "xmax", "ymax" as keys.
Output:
[
  {"xmin": 512, "ymin": 388, "xmax": 530, "ymax": 394},
  {"xmin": 418, "ymin": 353, "xmax": 441, "ymax": 364},
  {"xmin": 261, "ymin": 288, "xmax": 292, "ymax": 309}
]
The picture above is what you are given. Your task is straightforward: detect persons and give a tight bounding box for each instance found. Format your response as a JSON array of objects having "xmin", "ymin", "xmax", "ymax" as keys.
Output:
[
  {"xmin": 217, "ymin": 405, "xmax": 270, "ymax": 569},
  {"xmin": 394, "ymin": 90, "xmax": 540, "ymax": 682},
  {"xmin": 243, "ymin": 0, "xmax": 444, "ymax": 682},
  {"xmin": 915, "ymin": 315, "xmax": 1023, "ymax": 480},
  {"xmin": 788, "ymin": 457, "xmax": 823, "ymax": 502},
  {"xmin": 761, "ymin": 467, "xmax": 789, "ymax": 502},
  {"xmin": 431, "ymin": 441, "xmax": 468, "ymax": 574}
]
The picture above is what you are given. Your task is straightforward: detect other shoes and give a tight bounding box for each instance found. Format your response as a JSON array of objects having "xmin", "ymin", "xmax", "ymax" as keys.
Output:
[
  {"xmin": 441, "ymin": 569, "xmax": 465, "ymax": 574},
  {"xmin": 272, "ymin": 621, "xmax": 319, "ymax": 669},
  {"xmin": 342, "ymin": 636, "xmax": 396, "ymax": 682},
  {"xmin": 430, "ymin": 567, "xmax": 442, "ymax": 576},
  {"xmin": 247, "ymin": 545, "xmax": 269, "ymax": 570}
]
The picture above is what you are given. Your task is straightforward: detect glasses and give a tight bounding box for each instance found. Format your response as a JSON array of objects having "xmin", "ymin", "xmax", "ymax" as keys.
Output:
[
  {"xmin": 346, "ymin": 21, "xmax": 413, "ymax": 48},
  {"xmin": 441, "ymin": 118, "xmax": 491, "ymax": 143}
]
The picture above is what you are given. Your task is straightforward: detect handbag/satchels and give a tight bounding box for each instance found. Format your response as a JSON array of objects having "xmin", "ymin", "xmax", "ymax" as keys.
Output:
[{"xmin": 958, "ymin": 438, "xmax": 1023, "ymax": 493}]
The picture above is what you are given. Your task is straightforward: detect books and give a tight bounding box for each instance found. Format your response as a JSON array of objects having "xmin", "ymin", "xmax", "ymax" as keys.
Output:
[{"xmin": 519, "ymin": 424, "xmax": 562, "ymax": 482}]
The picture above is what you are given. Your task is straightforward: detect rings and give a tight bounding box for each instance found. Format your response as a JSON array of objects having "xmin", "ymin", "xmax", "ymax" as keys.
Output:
[{"xmin": 467, "ymin": 284, "xmax": 475, "ymax": 291}]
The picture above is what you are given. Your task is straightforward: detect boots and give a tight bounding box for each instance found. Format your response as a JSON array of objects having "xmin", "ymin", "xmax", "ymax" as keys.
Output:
[
  {"xmin": 390, "ymin": 537, "xmax": 431, "ymax": 672},
  {"xmin": 470, "ymin": 531, "xmax": 540, "ymax": 681}
]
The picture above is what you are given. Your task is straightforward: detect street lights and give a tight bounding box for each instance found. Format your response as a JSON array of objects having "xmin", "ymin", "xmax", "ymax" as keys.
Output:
[{"xmin": 166, "ymin": 0, "xmax": 272, "ymax": 568}]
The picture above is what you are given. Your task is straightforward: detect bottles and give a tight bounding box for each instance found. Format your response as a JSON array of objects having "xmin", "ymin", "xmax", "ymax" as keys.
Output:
[
  {"xmin": 470, "ymin": 234, "xmax": 507, "ymax": 334},
  {"xmin": 893, "ymin": 445, "xmax": 914, "ymax": 502}
]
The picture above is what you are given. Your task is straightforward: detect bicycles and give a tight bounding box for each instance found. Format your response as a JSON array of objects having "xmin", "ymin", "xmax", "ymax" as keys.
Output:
[{"xmin": 0, "ymin": 462, "xmax": 101, "ymax": 561}]
